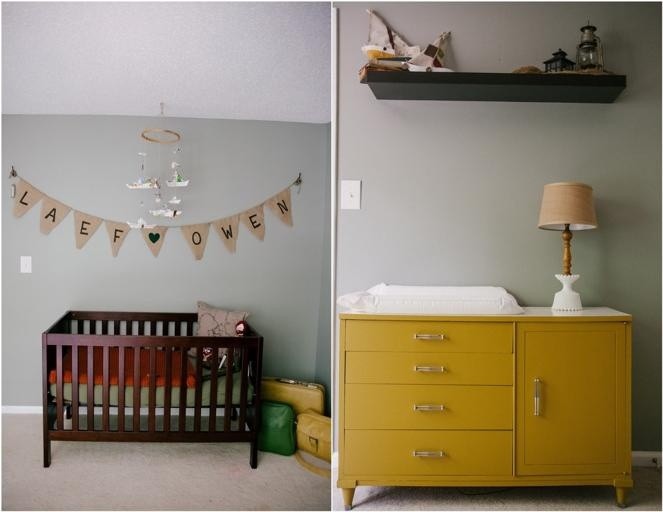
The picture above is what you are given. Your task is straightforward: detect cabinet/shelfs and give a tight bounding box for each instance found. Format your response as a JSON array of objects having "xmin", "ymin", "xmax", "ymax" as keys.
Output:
[{"xmin": 340, "ymin": 319, "xmax": 634, "ymax": 508}]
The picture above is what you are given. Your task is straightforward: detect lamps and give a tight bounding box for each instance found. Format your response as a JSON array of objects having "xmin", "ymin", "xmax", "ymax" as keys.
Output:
[{"xmin": 537, "ymin": 181, "xmax": 597, "ymax": 312}]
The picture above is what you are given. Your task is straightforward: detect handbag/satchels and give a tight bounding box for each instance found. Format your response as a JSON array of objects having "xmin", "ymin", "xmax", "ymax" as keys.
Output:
[
  {"xmin": 294, "ymin": 408, "xmax": 331, "ymax": 464},
  {"xmin": 257, "ymin": 400, "xmax": 297, "ymax": 456}
]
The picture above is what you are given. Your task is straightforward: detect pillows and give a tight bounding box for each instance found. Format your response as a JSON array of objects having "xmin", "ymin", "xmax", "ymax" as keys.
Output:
[
  {"xmin": 338, "ymin": 285, "xmax": 524, "ymax": 317},
  {"xmin": 188, "ymin": 300, "xmax": 249, "ymax": 368}
]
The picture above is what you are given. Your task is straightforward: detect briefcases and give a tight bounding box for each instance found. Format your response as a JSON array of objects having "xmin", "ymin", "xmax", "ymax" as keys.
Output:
[{"xmin": 260, "ymin": 375, "xmax": 326, "ymax": 416}]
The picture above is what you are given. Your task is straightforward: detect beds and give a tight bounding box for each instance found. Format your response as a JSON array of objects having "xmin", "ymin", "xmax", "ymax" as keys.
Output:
[{"xmin": 40, "ymin": 309, "xmax": 264, "ymax": 470}]
